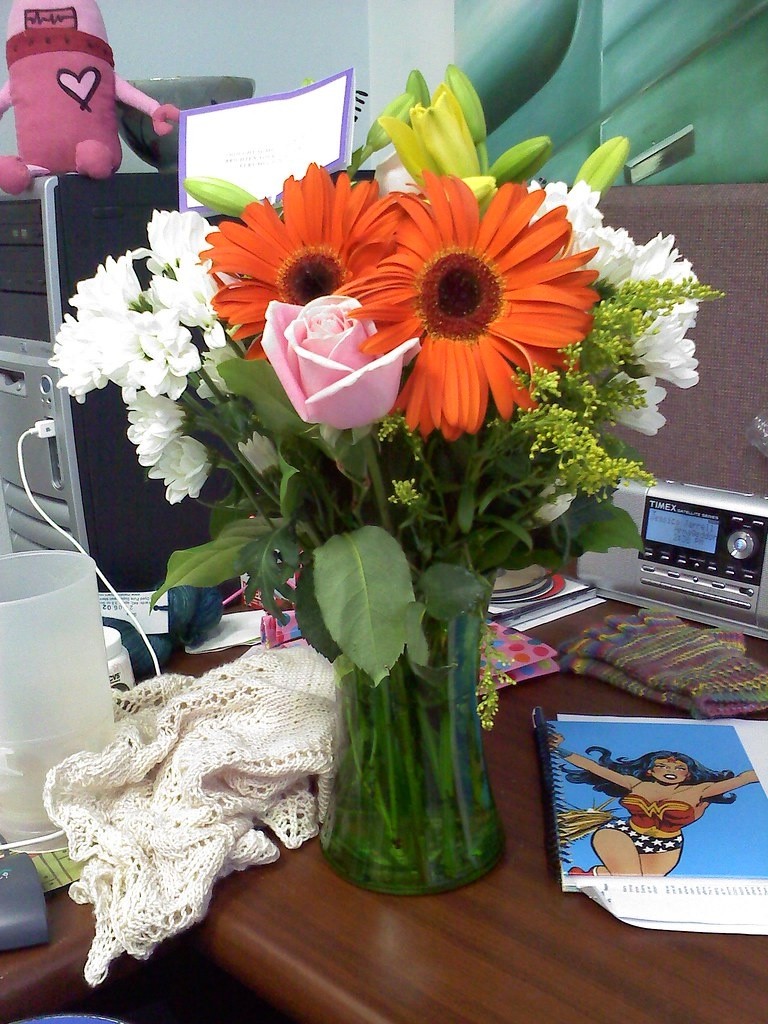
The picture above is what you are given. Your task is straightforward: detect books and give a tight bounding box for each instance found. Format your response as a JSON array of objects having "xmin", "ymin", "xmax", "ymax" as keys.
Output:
[
  {"xmin": 545, "ymin": 718, "xmax": 768, "ymax": 895},
  {"xmin": 482, "ymin": 568, "xmax": 603, "ymax": 634}
]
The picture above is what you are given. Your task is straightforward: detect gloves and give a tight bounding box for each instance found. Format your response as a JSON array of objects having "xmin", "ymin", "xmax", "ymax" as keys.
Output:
[{"xmin": 559, "ymin": 605, "xmax": 768, "ymax": 717}]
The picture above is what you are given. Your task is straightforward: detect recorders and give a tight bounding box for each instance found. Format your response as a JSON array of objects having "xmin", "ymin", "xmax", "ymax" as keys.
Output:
[{"xmin": 576, "ymin": 473, "xmax": 768, "ymax": 641}]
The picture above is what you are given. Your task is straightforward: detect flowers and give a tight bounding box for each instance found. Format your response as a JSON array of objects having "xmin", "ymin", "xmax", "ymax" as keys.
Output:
[{"xmin": 47, "ymin": 62, "xmax": 725, "ymax": 688}]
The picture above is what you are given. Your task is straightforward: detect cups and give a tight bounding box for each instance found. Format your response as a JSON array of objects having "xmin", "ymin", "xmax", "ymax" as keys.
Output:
[{"xmin": 0, "ymin": 550, "xmax": 112, "ymax": 852}]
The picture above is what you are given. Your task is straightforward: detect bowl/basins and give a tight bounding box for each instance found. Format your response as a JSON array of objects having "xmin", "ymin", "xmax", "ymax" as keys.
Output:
[{"xmin": 112, "ymin": 76, "xmax": 256, "ymax": 176}]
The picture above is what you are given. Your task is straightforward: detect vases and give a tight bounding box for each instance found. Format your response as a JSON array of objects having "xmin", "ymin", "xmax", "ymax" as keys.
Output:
[{"xmin": 320, "ymin": 592, "xmax": 502, "ymax": 899}]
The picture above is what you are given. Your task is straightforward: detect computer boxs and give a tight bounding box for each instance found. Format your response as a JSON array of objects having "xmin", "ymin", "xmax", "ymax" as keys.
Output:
[{"xmin": 0, "ymin": 168, "xmax": 242, "ymax": 594}]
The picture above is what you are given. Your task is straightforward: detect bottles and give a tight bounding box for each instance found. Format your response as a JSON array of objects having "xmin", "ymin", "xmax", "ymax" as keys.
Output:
[{"xmin": 100, "ymin": 622, "xmax": 135, "ymax": 691}]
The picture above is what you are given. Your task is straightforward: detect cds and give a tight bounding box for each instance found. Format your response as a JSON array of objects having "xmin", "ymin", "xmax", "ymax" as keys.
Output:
[{"xmin": 488, "ymin": 567, "xmax": 566, "ymax": 604}]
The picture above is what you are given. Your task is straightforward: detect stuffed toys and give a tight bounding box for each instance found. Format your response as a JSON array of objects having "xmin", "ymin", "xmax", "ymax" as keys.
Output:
[{"xmin": 1, "ymin": 1, "xmax": 181, "ymax": 197}]
